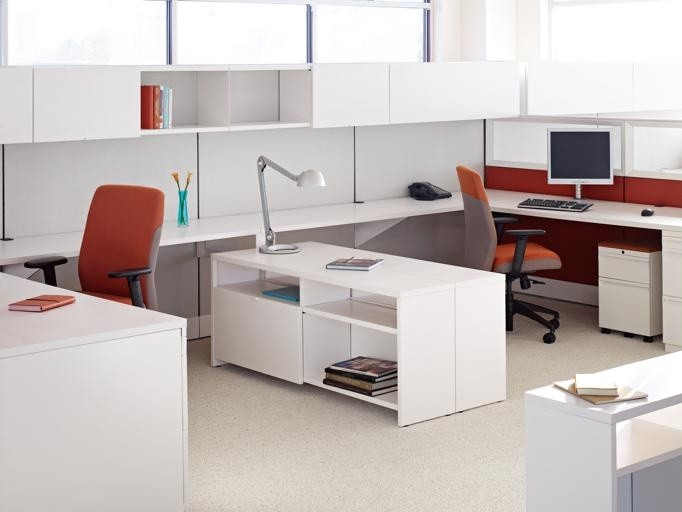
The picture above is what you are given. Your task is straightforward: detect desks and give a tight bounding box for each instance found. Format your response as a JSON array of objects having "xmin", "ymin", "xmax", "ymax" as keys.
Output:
[
  {"xmin": 0, "ymin": 273, "xmax": 187, "ymax": 512},
  {"xmin": 487, "ymin": 189, "xmax": 682, "ymax": 355},
  {"xmin": 0, "ymin": 188, "xmax": 487, "ymax": 340}
]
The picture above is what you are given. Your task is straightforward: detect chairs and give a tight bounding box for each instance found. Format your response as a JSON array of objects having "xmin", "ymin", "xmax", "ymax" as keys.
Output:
[
  {"xmin": 24, "ymin": 186, "xmax": 164, "ymax": 310},
  {"xmin": 453, "ymin": 167, "xmax": 561, "ymax": 345}
]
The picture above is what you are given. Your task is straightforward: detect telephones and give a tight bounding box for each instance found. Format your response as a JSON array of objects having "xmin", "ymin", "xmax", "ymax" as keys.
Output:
[{"xmin": 408, "ymin": 182, "xmax": 452, "ymax": 201}]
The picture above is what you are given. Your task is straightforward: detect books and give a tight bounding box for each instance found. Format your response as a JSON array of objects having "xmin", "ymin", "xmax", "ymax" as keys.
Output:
[
  {"xmin": 154, "ymin": 87, "xmax": 160, "ymax": 130},
  {"xmin": 163, "ymin": 88, "xmax": 169, "ymax": 129},
  {"xmin": 329, "ymin": 356, "xmax": 398, "ymax": 377},
  {"xmin": 326, "ymin": 257, "xmax": 386, "ymax": 271},
  {"xmin": 170, "ymin": 89, "xmax": 174, "ymax": 128},
  {"xmin": 324, "ymin": 373, "xmax": 397, "ymax": 391},
  {"xmin": 262, "ymin": 284, "xmax": 299, "ymax": 303},
  {"xmin": 325, "ymin": 366, "xmax": 398, "ymax": 383},
  {"xmin": 323, "ymin": 378, "xmax": 396, "ymax": 396},
  {"xmin": 160, "ymin": 86, "xmax": 164, "ymax": 128},
  {"xmin": 553, "ymin": 374, "xmax": 649, "ymax": 407},
  {"xmin": 140, "ymin": 85, "xmax": 154, "ymax": 129},
  {"xmin": 7, "ymin": 293, "xmax": 75, "ymax": 313},
  {"xmin": 576, "ymin": 372, "xmax": 618, "ymax": 396}
]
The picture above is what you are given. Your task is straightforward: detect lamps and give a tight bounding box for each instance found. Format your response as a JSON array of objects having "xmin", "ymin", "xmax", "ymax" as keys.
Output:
[{"xmin": 256, "ymin": 156, "xmax": 325, "ymax": 254}]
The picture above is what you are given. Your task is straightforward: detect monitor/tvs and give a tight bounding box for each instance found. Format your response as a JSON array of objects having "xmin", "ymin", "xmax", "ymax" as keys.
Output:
[{"xmin": 546, "ymin": 127, "xmax": 614, "ymax": 202}]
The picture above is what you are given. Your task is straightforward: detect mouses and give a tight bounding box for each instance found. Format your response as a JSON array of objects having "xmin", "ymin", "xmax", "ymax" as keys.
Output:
[{"xmin": 641, "ymin": 207, "xmax": 654, "ymax": 216}]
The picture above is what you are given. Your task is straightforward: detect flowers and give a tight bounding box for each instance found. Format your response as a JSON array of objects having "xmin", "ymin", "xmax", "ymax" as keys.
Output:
[{"xmin": 171, "ymin": 170, "xmax": 192, "ymax": 227}]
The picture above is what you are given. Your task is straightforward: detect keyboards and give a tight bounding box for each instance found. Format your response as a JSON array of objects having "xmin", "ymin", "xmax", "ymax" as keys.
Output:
[{"xmin": 517, "ymin": 197, "xmax": 593, "ymax": 212}]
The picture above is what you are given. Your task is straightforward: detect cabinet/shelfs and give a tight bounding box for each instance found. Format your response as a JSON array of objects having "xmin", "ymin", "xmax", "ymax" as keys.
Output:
[
  {"xmin": 521, "ymin": 352, "xmax": 681, "ymax": 512},
  {"xmin": 597, "ymin": 243, "xmax": 662, "ymax": 343},
  {"xmin": 0, "ymin": 65, "xmax": 534, "ymax": 144},
  {"xmin": 211, "ymin": 241, "xmax": 506, "ymax": 427}
]
